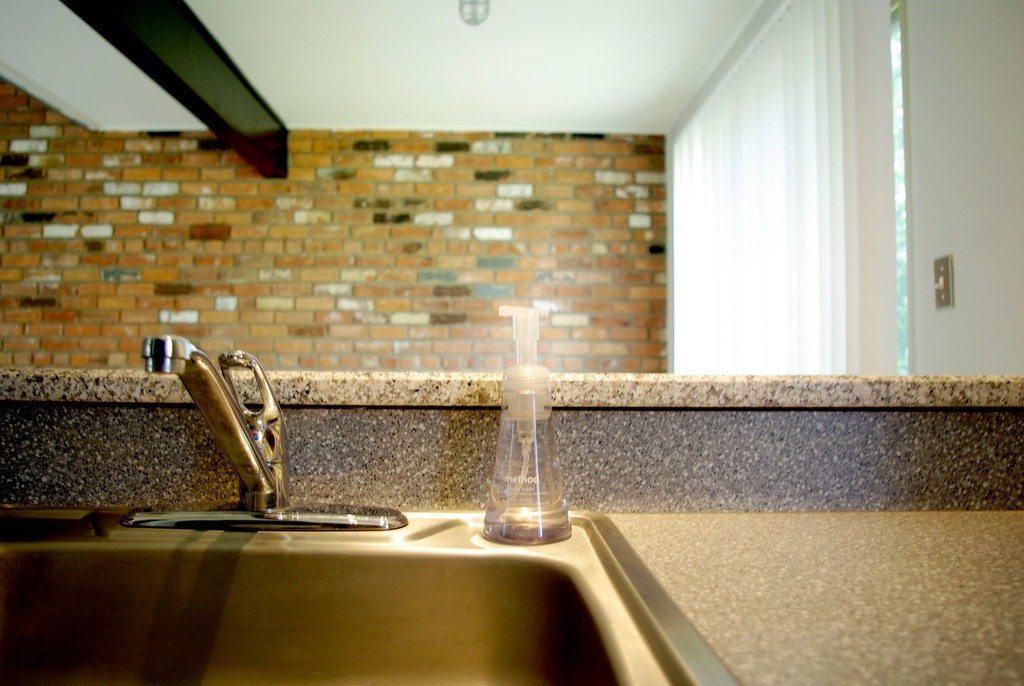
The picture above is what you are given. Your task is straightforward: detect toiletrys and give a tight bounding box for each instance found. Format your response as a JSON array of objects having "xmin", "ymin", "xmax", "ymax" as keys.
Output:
[{"xmin": 482, "ymin": 303, "xmax": 572, "ymax": 544}]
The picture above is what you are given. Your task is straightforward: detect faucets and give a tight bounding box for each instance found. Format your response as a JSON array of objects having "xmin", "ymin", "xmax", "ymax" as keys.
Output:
[{"xmin": 137, "ymin": 332, "xmax": 290, "ymax": 515}]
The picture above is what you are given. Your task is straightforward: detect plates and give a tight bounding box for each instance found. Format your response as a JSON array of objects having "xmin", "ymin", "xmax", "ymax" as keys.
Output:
[{"xmin": 0, "ymin": 503, "xmax": 130, "ymax": 541}]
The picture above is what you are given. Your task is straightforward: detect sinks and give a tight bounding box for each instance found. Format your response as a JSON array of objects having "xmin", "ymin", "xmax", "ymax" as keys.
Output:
[{"xmin": 3, "ymin": 501, "xmax": 740, "ymax": 685}]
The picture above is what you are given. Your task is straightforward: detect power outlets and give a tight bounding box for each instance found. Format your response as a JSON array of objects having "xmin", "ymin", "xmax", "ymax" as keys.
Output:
[{"xmin": 933, "ymin": 253, "xmax": 955, "ymax": 309}]
